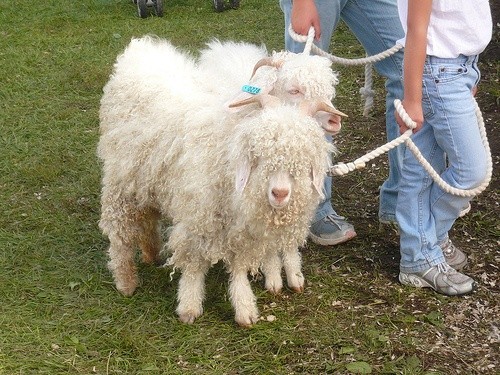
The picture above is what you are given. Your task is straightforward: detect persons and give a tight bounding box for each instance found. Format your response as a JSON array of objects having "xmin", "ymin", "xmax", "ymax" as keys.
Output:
[
  {"xmin": 394, "ymin": 0, "xmax": 493, "ymax": 296},
  {"xmin": 278, "ymin": 0, "xmax": 409, "ymax": 247}
]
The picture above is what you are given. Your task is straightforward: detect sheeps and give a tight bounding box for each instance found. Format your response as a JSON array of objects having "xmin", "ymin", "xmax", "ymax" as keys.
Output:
[
  {"xmin": 103, "ymin": 33, "xmax": 341, "ymax": 135},
  {"xmin": 95, "ymin": 93, "xmax": 349, "ymax": 330}
]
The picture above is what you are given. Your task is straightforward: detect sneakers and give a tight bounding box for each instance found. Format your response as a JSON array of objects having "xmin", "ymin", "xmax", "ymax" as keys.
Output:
[
  {"xmin": 308, "ymin": 213, "xmax": 357, "ymax": 246},
  {"xmin": 436, "ymin": 234, "xmax": 467, "ymax": 270},
  {"xmin": 378, "ymin": 213, "xmax": 399, "ymax": 225},
  {"xmin": 399, "ymin": 261, "xmax": 476, "ymax": 296}
]
